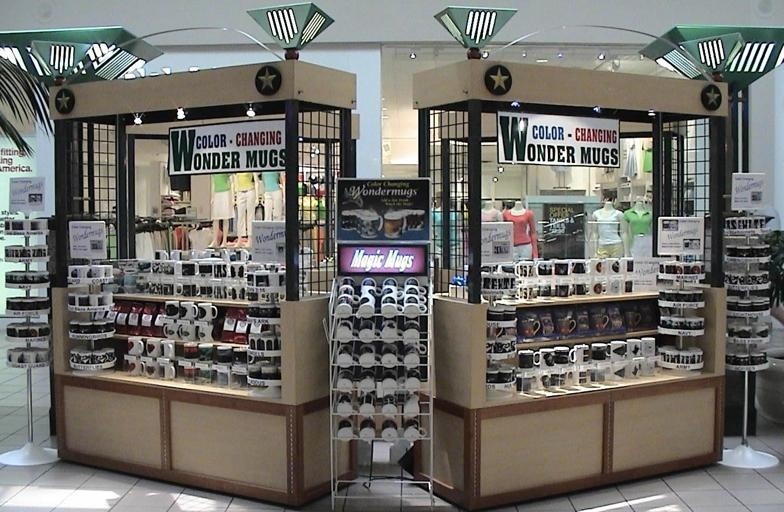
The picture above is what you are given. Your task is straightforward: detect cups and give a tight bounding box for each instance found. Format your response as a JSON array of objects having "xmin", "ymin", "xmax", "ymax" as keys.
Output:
[
  {"xmin": 339, "ymin": 207, "xmax": 426, "ymax": 240},
  {"xmin": 518, "ymin": 337, "xmax": 658, "ymax": 394},
  {"xmin": 519, "ymin": 307, "xmax": 642, "ymax": 338},
  {"xmin": 121, "ymin": 249, "xmax": 312, "ymax": 388},
  {"xmin": 515, "ymin": 256, "xmax": 635, "ymax": 302},
  {"xmin": 67, "ymin": 263, "xmax": 117, "ymax": 372},
  {"xmin": 481, "ymin": 265, "xmax": 516, "ymax": 400},
  {"xmin": 657, "ymin": 259, "xmax": 707, "ymax": 372},
  {"xmin": 335, "ymin": 276, "xmax": 427, "ymax": 443},
  {"xmin": 3, "ymin": 217, "xmax": 53, "ymax": 366},
  {"xmin": 725, "ymin": 216, "xmax": 772, "ymax": 372}
]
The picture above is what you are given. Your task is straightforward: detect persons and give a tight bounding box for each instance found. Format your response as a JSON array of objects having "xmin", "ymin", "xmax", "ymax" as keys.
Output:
[
  {"xmin": 592, "ymin": 202, "xmax": 653, "ymax": 258},
  {"xmin": 168, "ymin": 172, "xmax": 286, "ymax": 248},
  {"xmin": 478, "ymin": 202, "xmax": 538, "ymax": 262}
]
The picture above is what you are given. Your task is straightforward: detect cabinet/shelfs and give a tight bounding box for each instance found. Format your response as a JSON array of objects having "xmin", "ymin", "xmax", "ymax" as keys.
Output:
[
  {"xmin": 414, "ymin": 291, "xmax": 728, "ymax": 511},
  {"xmin": 0, "ymin": 218, "xmax": 59, "ymax": 467},
  {"xmin": 47, "ymin": 282, "xmax": 360, "ymax": 510},
  {"xmin": 325, "ymin": 277, "xmax": 442, "ymax": 511},
  {"xmin": 718, "ymin": 225, "xmax": 782, "ymax": 478}
]
